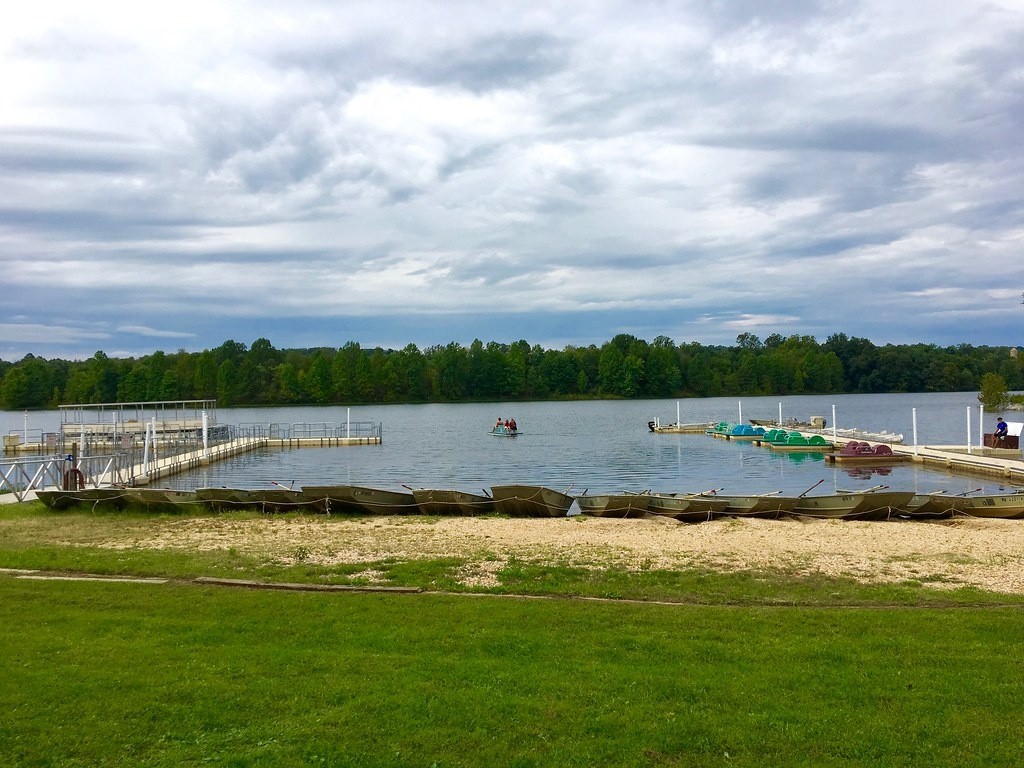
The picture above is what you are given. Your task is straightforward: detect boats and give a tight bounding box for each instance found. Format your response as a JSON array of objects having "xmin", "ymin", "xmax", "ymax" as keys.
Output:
[
  {"xmin": 488, "ymin": 422, "xmax": 524, "ymax": 438},
  {"xmin": 824, "ymin": 440, "xmax": 911, "ymax": 463},
  {"xmin": 705, "ymin": 420, "xmax": 834, "ymax": 450}
]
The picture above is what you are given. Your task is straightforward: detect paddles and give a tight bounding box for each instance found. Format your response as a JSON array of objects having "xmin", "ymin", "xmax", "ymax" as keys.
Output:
[
  {"xmin": 581, "ymin": 488, "xmax": 588, "ymax": 496},
  {"xmin": 954, "ymin": 488, "xmax": 982, "ymax": 496},
  {"xmin": 685, "ymin": 487, "xmax": 724, "ymax": 499},
  {"xmin": 798, "ymin": 479, "xmax": 824, "ymax": 498},
  {"xmin": 927, "ymin": 490, "xmax": 947, "ymax": 495},
  {"xmin": 270, "ymin": 480, "xmax": 294, "ymax": 490},
  {"xmin": 482, "ymin": 488, "xmax": 490, "ymax": 498},
  {"xmin": 111, "ymin": 482, "xmax": 124, "ymax": 489},
  {"xmin": 857, "ymin": 484, "xmax": 889, "ymax": 494},
  {"xmin": 639, "ymin": 489, "xmax": 652, "ymax": 495},
  {"xmin": 401, "ymin": 484, "xmax": 414, "ymax": 490},
  {"xmin": 564, "ymin": 484, "xmax": 574, "ymax": 494},
  {"xmin": 762, "ymin": 491, "xmax": 783, "ymax": 496}
]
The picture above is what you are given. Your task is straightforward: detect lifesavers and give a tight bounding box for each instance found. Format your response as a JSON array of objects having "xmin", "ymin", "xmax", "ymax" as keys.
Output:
[{"xmin": 63, "ymin": 468, "xmax": 85, "ymax": 491}]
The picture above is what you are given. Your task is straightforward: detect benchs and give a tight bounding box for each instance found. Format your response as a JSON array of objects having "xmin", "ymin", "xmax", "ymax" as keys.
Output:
[{"xmin": 984, "ymin": 421, "xmax": 1024, "ymax": 449}]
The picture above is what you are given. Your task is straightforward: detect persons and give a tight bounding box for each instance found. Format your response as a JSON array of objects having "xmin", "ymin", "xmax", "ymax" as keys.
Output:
[
  {"xmin": 990, "ymin": 418, "xmax": 1007, "ymax": 449},
  {"xmin": 496, "ymin": 418, "xmax": 518, "ymax": 433}
]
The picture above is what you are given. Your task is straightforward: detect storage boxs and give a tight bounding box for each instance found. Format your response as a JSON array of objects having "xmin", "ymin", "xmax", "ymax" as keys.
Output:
[{"xmin": 2, "ymin": 434, "xmax": 19, "ymax": 446}]
